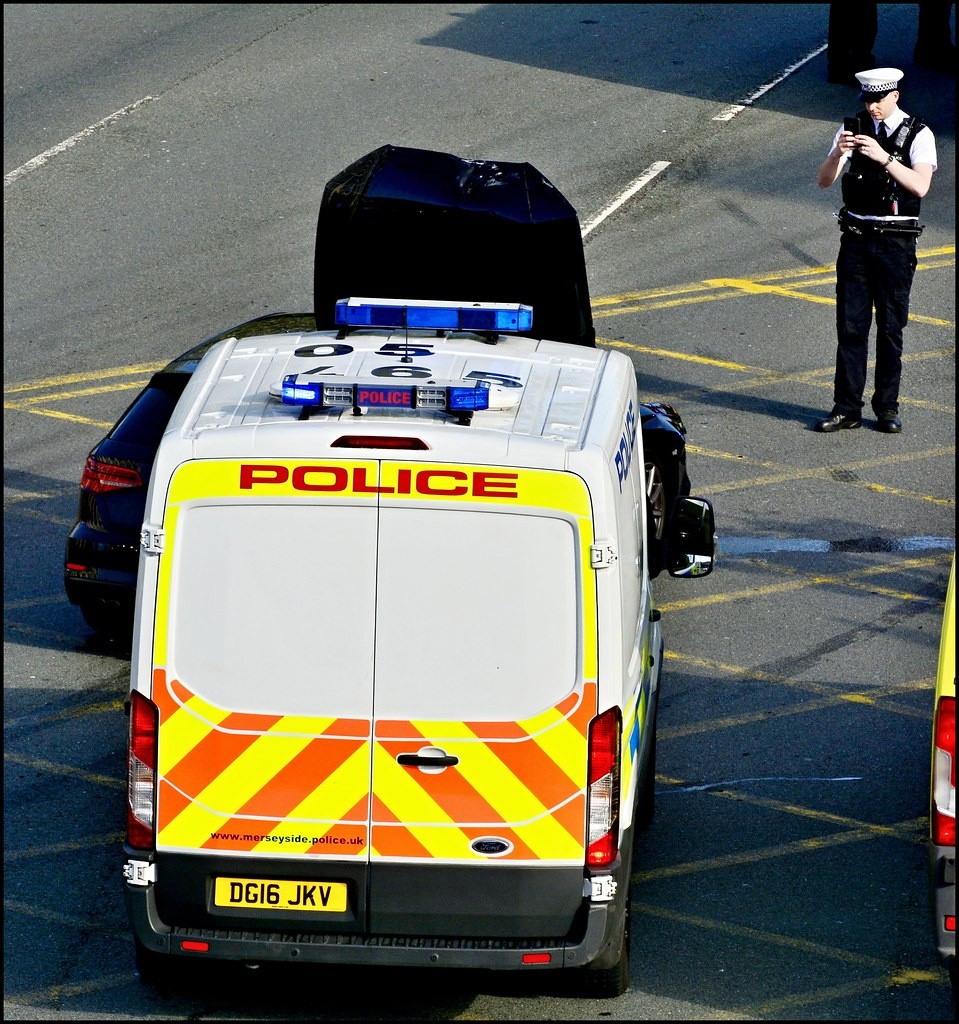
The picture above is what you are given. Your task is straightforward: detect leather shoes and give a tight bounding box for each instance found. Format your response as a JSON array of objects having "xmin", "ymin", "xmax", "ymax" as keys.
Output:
[
  {"xmin": 877, "ymin": 410, "xmax": 903, "ymax": 432},
  {"xmin": 816, "ymin": 411, "xmax": 862, "ymax": 432}
]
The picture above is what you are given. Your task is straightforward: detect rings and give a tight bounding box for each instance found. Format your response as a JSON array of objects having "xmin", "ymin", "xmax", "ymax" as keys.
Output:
[{"xmin": 864, "ymin": 146, "xmax": 866, "ymax": 150}]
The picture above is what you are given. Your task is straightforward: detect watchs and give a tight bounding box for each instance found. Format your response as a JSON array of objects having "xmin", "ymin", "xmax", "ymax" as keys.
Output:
[{"xmin": 881, "ymin": 155, "xmax": 893, "ymax": 167}]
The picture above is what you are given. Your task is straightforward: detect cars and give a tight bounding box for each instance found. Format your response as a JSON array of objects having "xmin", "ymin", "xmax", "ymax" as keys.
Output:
[{"xmin": 60, "ymin": 140, "xmax": 689, "ymax": 638}]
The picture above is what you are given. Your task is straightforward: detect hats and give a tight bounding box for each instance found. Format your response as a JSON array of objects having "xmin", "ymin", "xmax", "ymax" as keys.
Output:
[{"xmin": 855, "ymin": 68, "xmax": 904, "ymax": 103}]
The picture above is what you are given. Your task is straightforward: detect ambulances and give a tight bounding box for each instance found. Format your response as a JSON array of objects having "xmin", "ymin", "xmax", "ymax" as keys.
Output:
[{"xmin": 121, "ymin": 326, "xmax": 667, "ymax": 997}]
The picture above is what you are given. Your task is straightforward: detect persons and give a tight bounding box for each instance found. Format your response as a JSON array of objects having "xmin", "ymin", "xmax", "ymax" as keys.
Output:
[{"xmin": 815, "ymin": 67, "xmax": 938, "ymax": 432}]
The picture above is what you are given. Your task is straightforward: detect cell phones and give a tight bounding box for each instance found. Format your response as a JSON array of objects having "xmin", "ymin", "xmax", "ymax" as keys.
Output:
[{"xmin": 843, "ymin": 115, "xmax": 860, "ymax": 139}]
